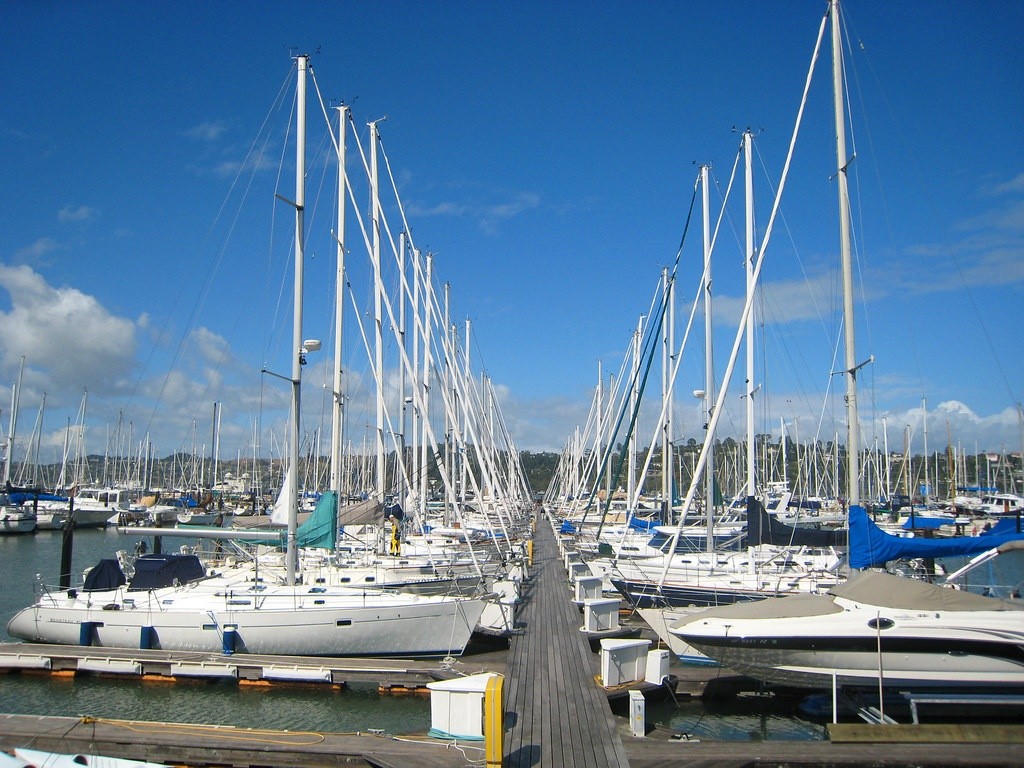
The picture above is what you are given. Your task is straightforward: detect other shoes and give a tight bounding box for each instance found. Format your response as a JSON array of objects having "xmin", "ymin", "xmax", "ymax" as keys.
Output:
[{"xmin": 390, "ymin": 553, "xmax": 400, "ymax": 557}]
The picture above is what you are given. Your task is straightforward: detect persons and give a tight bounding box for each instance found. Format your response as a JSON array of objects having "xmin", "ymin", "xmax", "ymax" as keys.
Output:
[{"xmin": 389, "ymin": 515, "xmax": 401, "ymax": 556}]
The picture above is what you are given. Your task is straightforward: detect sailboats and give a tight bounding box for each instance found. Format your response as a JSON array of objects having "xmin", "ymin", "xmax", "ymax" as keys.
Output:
[{"xmin": 2, "ymin": 1, "xmax": 1024, "ymax": 699}]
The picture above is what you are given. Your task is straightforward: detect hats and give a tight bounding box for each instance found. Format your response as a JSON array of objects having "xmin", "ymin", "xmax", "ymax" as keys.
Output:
[{"xmin": 389, "ymin": 515, "xmax": 394, "ymax": 518}]
